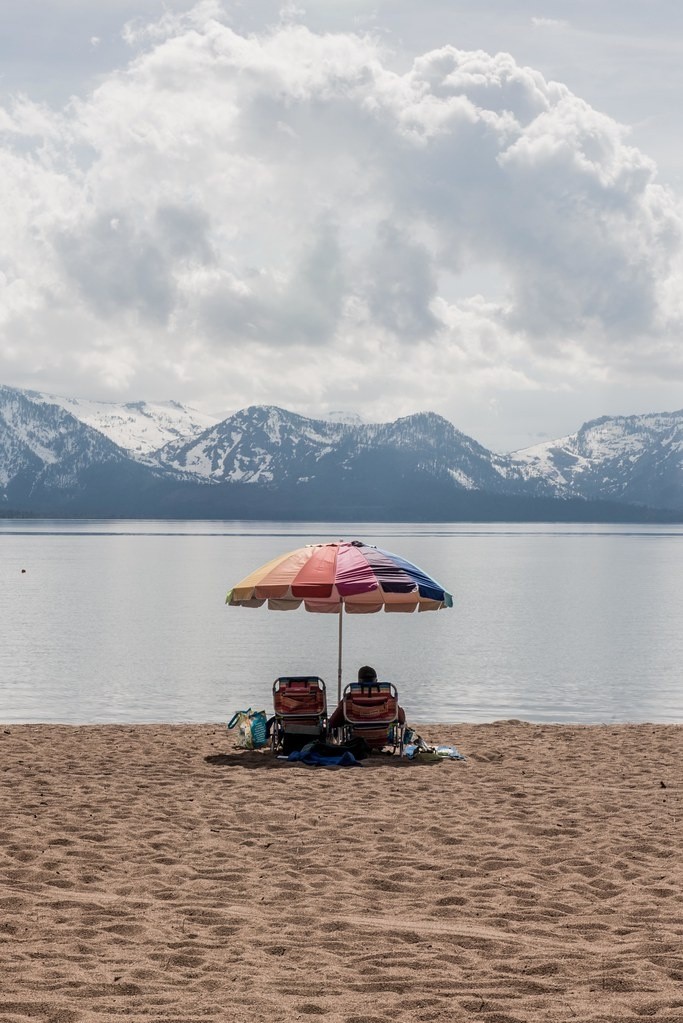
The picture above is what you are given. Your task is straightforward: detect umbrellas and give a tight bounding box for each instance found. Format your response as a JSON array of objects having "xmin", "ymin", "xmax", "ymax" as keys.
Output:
[{"xmin": 225, "ymin": 539, "xmax": 453, "ymax": 703}]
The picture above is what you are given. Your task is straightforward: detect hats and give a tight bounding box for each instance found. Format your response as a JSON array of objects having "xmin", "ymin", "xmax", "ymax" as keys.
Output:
[{"xmin": 358, "ymin": 666, "xmax": 377, "ymax": 683}]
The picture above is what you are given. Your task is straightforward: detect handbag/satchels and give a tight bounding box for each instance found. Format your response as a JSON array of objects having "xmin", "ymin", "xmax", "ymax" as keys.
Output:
[
  {"xmin": 249, "ymin": 710, "xmax": 270, "ymax": 749},
  {"xmin": 228, "ymin": 707, "xmax": 254, "ymax": 750}
]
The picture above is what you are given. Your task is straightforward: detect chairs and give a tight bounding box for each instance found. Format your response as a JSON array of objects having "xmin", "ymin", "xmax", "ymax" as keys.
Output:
[
  {"xmin": 341, "ymin": 681, "xmax": 408, "ymax": 758},
  {"xmin": 268, "ymin": 676, "xmax": 330, "ymax": 770}
]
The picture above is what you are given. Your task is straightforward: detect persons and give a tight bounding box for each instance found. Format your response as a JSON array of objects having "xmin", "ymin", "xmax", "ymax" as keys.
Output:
[{"xmin": 329, "ymin": 666, "xmax": 405, "ymax": 744}]
